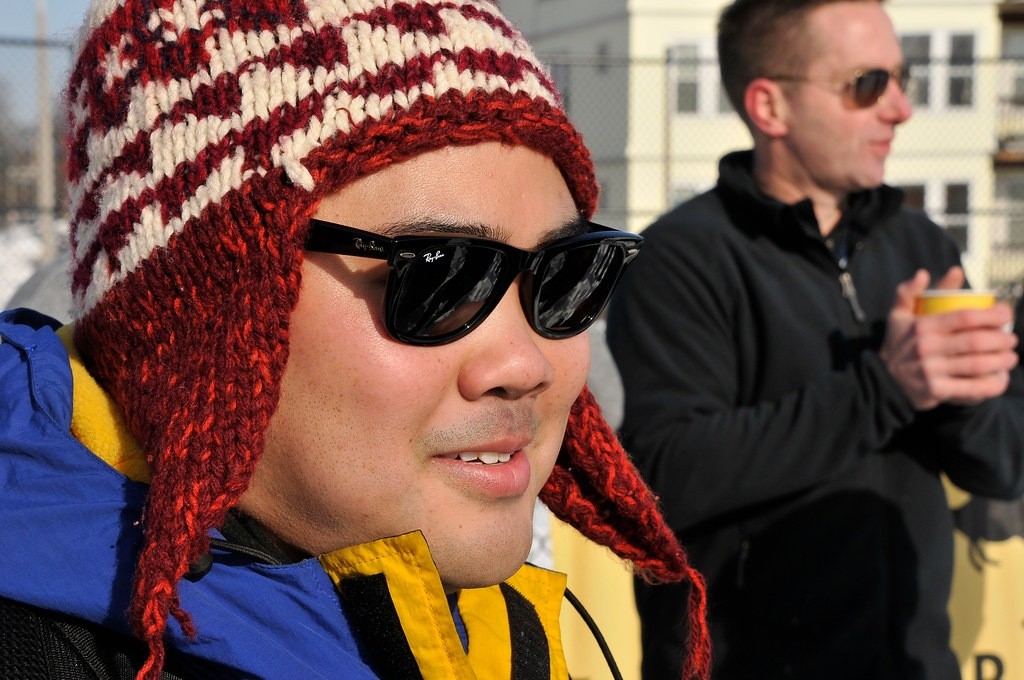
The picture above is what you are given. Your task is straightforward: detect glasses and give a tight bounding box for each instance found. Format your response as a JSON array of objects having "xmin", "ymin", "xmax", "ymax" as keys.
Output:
[
  {"xmin": 303, "ymin": 219, "xmax": 646, "ymax": 347},
  {"xmin": 758, "ymin": 57, "xmax": 912, "ymax": 110}
]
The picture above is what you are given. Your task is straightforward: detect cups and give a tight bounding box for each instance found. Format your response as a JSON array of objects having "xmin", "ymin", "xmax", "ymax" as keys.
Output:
[{"xmin": 913, "ymin": 288, "xmax": 996, "ymax": 315}]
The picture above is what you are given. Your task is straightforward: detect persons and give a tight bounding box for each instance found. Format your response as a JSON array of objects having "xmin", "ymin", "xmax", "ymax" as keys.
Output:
[
  {"xmin": 0, "ymin": 0, "xmax": 717, "ymax": 680},
  {"xmin": 604, "ymin": 0, "xmax": 1024, "ymax": 680}
]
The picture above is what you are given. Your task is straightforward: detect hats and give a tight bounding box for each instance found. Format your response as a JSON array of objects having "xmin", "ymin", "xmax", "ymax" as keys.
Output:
[{"xmin": 62, "ymin": 1, "xmax": 711, "ymax": 680}]
